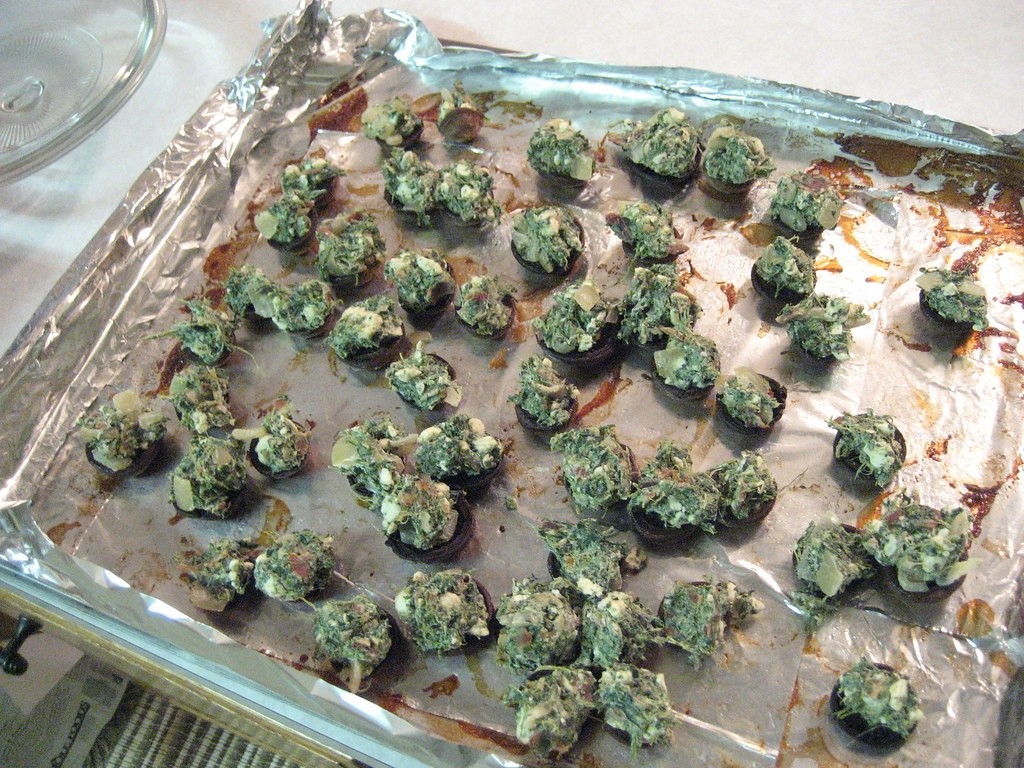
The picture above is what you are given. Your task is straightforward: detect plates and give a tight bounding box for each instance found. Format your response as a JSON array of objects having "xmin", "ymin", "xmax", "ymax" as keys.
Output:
[{"xmin": 2, "ymin": 1, "xmax": 169, "ymax": 184}]
